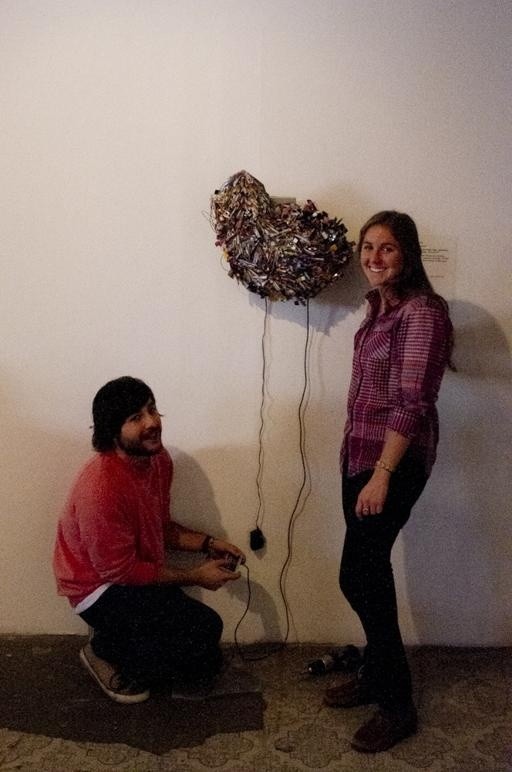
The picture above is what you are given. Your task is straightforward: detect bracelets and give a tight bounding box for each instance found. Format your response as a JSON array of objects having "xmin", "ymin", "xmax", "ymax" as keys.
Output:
[
  {"xmin": 375, "ymin": 460, "xmax": 395, "ymax": 473},
  {"xmin": 201, "ymin": 535, "xmax": 212, "ymax": 554}
]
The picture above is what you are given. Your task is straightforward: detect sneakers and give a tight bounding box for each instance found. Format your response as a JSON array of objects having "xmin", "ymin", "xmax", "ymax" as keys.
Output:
[
  {"xmin": 74, "ymin": 641, "xmax": 149, "ymax": 704},
  {"xmin": 326, "ymin": 672, "xmax": 379, "ymax": 710},
  {"xmin": 352, "ymin": 704, "xmax": 418, "ymax": 756}
]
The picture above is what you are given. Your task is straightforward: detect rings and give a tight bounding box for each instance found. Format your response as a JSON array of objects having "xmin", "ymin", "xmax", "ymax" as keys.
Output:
[{"xmin": 363, "ymin": 508, "xmax": 370, "ymax": 515}]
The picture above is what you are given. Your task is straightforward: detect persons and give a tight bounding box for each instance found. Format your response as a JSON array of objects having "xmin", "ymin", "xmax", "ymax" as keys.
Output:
[
  {"xmin": 322, "ymin": 212, "xmax": 453, "ymax": 754},
  {"xmin": 53, "ymin": 376, "xmax": 246, "ymax": 706}
]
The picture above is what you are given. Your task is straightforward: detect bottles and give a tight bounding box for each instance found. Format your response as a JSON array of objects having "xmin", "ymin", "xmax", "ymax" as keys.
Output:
[{"xmin": 300, "ymin": 645, "xmax": 361, "ymax": 675}]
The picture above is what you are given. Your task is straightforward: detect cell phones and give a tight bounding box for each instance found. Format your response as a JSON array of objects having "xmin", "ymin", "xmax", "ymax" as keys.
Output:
[{"xmin": 213, "ymin": 549, "xmax": 241, "ymax": 574}]
